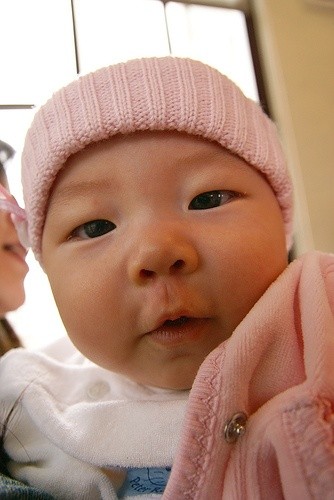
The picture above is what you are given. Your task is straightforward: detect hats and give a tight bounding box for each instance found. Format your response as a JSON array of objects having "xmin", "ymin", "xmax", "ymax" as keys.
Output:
[{"xmin": 0, "ymin": 55, "xmax": 296, "ymax": 276}]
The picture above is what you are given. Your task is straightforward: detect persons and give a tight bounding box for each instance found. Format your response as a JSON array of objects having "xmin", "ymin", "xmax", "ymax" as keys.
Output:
[
  {"xmin": 0, "ymin": 56, "xmax": 333, "ymax": 500},
  {"xmin": 0, "ymin": 139, "xmax": 52, "ymax": 499}
]
